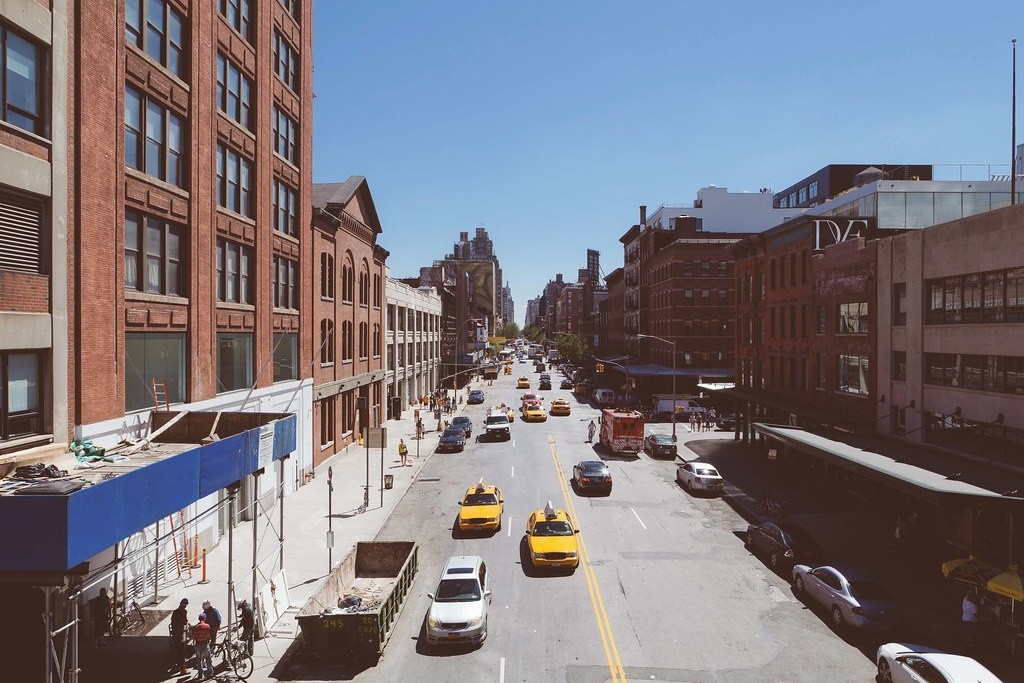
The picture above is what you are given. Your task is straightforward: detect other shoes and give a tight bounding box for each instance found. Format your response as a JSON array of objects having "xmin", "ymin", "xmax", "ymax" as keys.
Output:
[
  {"xmin": 100, "ymin": 640, "xmax": 107, "ymax": 645},
  {"xmin": 208, "ymin": 673, "xmax": 214, "ymax": 676},
  {"xmin": 180, "ymin": 668, "xmax": 191, "ymax": 675},
  {"xmin": 250, "ymin": 653, "xmax": 253, "ymax": 656},
  {"xmin": 198, "ymin": 674, "xmax": 202, "ymax": 679},
  {"xmin": 94, "ymin": 644, "xmax": 99, "ymax": 649}
]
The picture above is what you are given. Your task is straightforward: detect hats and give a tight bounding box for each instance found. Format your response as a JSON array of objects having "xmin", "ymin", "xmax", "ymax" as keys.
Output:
[
  {"xmin": 199, "ymin": 613, "xmax": 206, "ymax": 619},
  {"xmin": 181, "ymin": 599, "xmax": 188, "ymax": 604}
]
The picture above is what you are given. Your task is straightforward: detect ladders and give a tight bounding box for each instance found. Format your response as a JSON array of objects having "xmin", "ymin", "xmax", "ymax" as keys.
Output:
[{"xmin": 151, "ymin": 376, "xmax": 191, "ymax": 578}]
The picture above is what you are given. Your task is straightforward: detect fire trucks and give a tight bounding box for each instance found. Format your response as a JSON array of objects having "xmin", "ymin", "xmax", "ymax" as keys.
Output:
[{"xmin": 597, "ymin": 407, "xmax": 645, "ymax": 454}]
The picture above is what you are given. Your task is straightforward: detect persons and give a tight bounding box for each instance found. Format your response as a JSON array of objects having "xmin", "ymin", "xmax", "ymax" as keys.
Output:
[
  {"xmin": 91, "ymin": 588, "xmax": 112, "ymax": 649},
  {"xmin": 418, "ymin": 387, "xmax": 455, "ymax": 413},
  {"xmin": 416, "ymin": 418, "xmax": 426, "ymax": 439},
  {"xmin": 614, "ymin": 391, "xmax": 635, "ymax": 407},
  {"xmin": 587, "ymin": 421, "xmax": 596, "ymax": 441},
  {"xmin": 398, "ymin": 438, "xmax": 408, "ymax": 467},
  {"xmin": 961, "ymin": 590, "xmax": 989, "ymax": 652},
  {"xmin": 549, "ymin": 363, "xmax": 552, "ymax": 370},
  {"xmin": 689, "ymin": 407, "xmax": 716, "ymax": 433},
  {"xmin": 895, "ymin": 512, "xmax": 921, "ymax": 555},
  {"xmin": 171, "ymin": 599, "xmax": 255, "ymax": 680}
]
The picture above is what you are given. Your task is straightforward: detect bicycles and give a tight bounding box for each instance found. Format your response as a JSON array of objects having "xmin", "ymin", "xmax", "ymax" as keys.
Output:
[
  {"xmin": 106, "ymin": 596, "xmax": 145, "ymax": 635},
  {"xmin": 209, "ymin": 631, "xmax": 253, "ymax": 679}
]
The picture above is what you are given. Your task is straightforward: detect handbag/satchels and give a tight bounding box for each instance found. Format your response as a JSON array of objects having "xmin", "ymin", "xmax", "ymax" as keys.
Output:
[{"xmin": 404, "ymin": 450, "xmax": 408, "ymax": 455}]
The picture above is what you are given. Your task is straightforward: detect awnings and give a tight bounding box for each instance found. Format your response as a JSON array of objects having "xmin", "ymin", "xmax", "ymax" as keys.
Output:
[
  {"xmin": 442, "ymin": 349, "xmax": 483, "ymax": 363},
  {"xmin": 595, "ymin": 352, "xmax": 735, "ymax": 377},
  {"xmin": 466, "ymin": 341, "xmax": 489, "ymax": 349},
  {"xmin": 0, "ymin": 411, "xmax": 296, "ymax": 569},
  {"xmin": 749, "ymin": 422, "xmax": 1023, "ymax": 500}
]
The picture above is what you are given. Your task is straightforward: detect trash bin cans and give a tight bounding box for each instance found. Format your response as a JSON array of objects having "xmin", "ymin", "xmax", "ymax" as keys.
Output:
[{"xmin": 385, "ymin": 475, "xmax": 393, "ymax": 489}]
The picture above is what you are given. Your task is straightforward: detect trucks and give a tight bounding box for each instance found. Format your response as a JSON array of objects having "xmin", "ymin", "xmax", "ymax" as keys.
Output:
[{"xmin": 656, "ymin": 399, "xmax": 703, "ymax": 421}]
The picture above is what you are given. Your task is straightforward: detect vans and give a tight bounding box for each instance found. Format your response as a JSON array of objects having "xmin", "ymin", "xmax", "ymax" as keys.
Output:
[
  {"xmin": 595, "ymin": 388, "xmax": 616, "ymax": 404},
  {"xmin": 483, "ymin": 409, "xmax": 511, "ymax": 441}
]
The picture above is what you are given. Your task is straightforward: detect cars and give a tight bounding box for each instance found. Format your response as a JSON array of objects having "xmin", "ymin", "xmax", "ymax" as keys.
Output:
[
  {"xmin": 645, "ymin": 434, "xmax": 678, "ymax": 458},
  {"xmin": 794, "ymin": 560, "xmax": 908, "ymax": 632},
  {"xmin": 521, "ymin": 393, "xmax": 542, "ymax": 406},
  {"xmin": 747, "ymin": 521, "xmax": 819, "ymax": 568},
  {"xmin": 499, "ymin": 339, "xmax": 593, "ymax": 393},
  {"xmin": 458, "ymin": 477, "xmax": 504, "ymax": 531},
  {"xmin": 469, "ymin": 390, "xmax": 485, "ymax": 404},
  {"xmin": 677, "ymin": 462, "xmax": 724, "ymax": 494},
  {"xmin": 523, "ymin": 404, "xmax": 547, "ymax": 421},
  {"xmin": 449, "ymin": 416, "xmax": 473, "ymax": 438},
  {"xmin": 876, "ymin": 640, "xmax": 1003, "ymax": 683},
  {"xmin": 572, "ymin": 460, "xmax": 613, "ymax": 494},
  {"xmin": 525, "ymin": 501, "xmax": 581, "ymax": 568},
  {"xmin": 496, "ymin": 404, "xmax": 515, "ymax": 422},
  {"xmin": 551, "ymin": 398, "xmax": 571, "ymax": 415},
  {"xmin": 437, "ymin": 427, "xmax": 467, "ymax": 451},
  {"xmin": 716, "ymin": 413, "xmax": 744, "ymax": 430}
]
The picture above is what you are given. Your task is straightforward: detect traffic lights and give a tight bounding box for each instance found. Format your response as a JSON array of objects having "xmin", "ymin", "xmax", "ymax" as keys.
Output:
[
  {"xmin": 596, "ymin": 365, "xmax": 601, "ymax": 373},
  {"xmin": 600, "ymin": 365, "xmax": 604, "ymax": 373}
]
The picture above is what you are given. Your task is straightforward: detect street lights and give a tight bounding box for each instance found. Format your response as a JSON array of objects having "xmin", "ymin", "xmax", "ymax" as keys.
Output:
[
  {"xmin": 454, "ymin": 318, "xmax": 484, "ymax": 404},
  {"xmin": 636, "ymin": 333, "xmax": 677, "ymax": 434}
]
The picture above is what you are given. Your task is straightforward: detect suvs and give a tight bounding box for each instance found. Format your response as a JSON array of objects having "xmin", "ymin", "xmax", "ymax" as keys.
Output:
[{"xmin": 424, "ymin": 555, "xmax": 491, "ymax": 647}]
